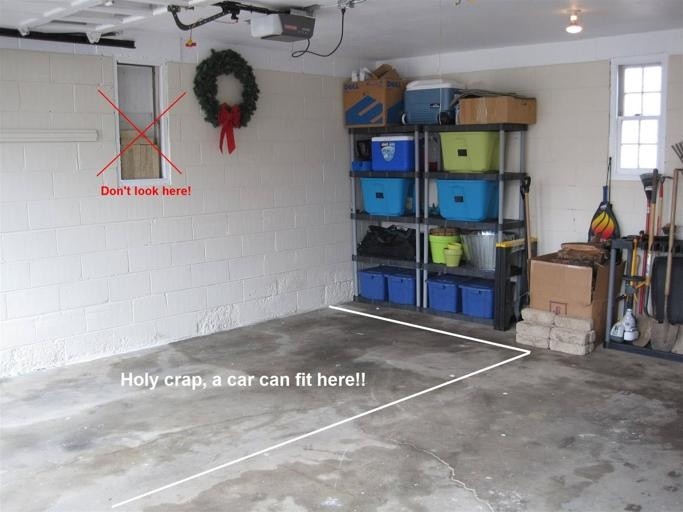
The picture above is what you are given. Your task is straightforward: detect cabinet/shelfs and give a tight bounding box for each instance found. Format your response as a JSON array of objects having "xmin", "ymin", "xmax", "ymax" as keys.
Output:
[{"xmin": 348, "ymin": 123, "xmax": 529, "ymax": 331}]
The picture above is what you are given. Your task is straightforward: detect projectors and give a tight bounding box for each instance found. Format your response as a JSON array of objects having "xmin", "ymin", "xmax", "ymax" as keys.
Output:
[{"xmin": 251, "ymin": 13, "xmax": 316, "ymax": 43}]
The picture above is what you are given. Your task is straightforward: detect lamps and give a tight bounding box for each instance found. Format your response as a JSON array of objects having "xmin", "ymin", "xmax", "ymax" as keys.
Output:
[{"xmin": 565, "ymin": 9, "xmax": 583, "ymax": 34}]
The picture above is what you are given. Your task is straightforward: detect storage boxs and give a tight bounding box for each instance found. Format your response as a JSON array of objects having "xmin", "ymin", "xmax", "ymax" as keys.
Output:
[
  {"xmin": 389, "ymin": 270, "xmax": 436, "ymax": 305},
  {"xmin": 460, "ymin": 278, "xmax": 515, "ymax": 318},
  {"xmin": 439, "ymin": 132, "xmax": 498, "ymax": 173},
  {"xmin": 343, "ymin": 64, "xmax": 409, "ymax": 126},
  {"xmin": 528, "ymin": 243, "xmax": 626, "ymax": 347},
  {"xmin": 425, "ymin": 273, "xmax": 473, "ymax": 313},
  {"xmin": 459, "ymin": 96, "xmax": 536, "ymax": 125},
  {"xmin": 436, "ymin": 180, "xmax": 499, "ymax": 222},
  {"xmin": 360, "ymin": 178, "xmax": 415, "ymax": 216},
  {"xmin": 359, "ymin": 266, "xmax": 401, "ymax": 301},
  {"xmin": 372, "ymin": 134, "xmax": 423, "ymax": 171},
  {"xmin": 404, "ymin": 79, "xmax": 460, "ymax": 124}
]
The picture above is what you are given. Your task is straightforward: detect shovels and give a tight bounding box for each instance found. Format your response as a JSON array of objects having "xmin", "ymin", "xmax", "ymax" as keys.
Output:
[
  {"xmin": 520, "ymin": 176, "xmax": 532, "ymax": 308},
  {"xmin": 630, "ymin": 168, "xmax": 683, "ymax": 355}
]
